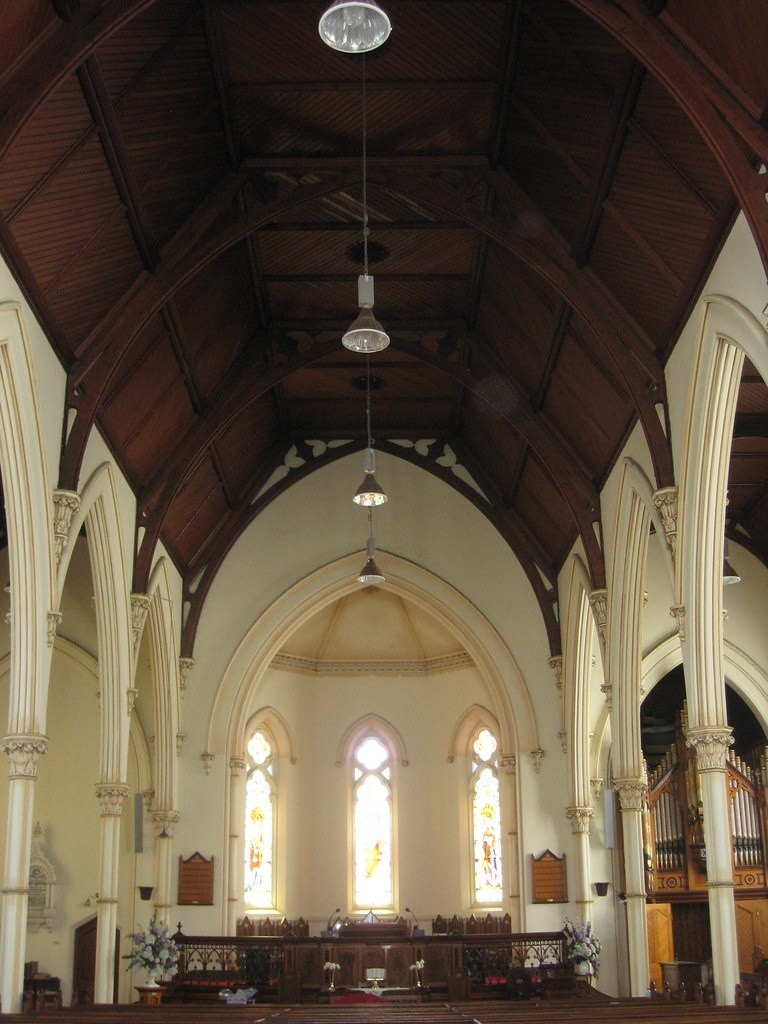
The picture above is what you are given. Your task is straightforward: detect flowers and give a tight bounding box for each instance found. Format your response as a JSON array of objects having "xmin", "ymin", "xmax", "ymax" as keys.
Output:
[
  {"xmin": 562, "ymin": 914, "xmax": 602, "ymax": 980},
  {"xmin": 122, "ymin": 909, "xmax": 181, "ymax": 976}
]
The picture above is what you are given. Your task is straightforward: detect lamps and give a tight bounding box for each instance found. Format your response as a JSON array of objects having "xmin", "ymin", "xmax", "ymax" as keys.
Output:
[
  {"xmin": 84, "ymin": 893, "xmax": 98, "ymax": 906},
  {"xmin": 342, "ymin": 51, "xmax": 390, "ymax": 354},
  {"xmin": 610, "ymin": 883, "xmax": 627, "ymax": 903},
  {"xmin": 318, "ymin": 0, "xmax": 392, "ymax": 53},
  {"xmin": 157, "ymin": 822, "xmax": 171, "ymax": 840},
  {"xmin": 723, "ymin": 538, "xmax": 741, "ymax": 586},
  {"xmin": 352, "ymin": 354, "xmax": 388, "ymax": 506},
  {"xmin": 356, "ymin": 508, "xmax": 386, "ymax": 584}
]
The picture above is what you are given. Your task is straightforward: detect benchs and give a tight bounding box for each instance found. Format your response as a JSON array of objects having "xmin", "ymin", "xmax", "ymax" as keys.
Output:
[{"xmin": 0, "ymin": 982, "xmax": 768, "ymax": 1024}]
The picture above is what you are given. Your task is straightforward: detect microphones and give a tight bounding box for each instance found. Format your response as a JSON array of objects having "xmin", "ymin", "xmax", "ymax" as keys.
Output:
[
  {"xmin": 405, "ymin": 908, "xmax": 419, "ymax": 930},
  {"xmin": 327, "ymin": 909, "xmax": 340, "ymax": 932}
]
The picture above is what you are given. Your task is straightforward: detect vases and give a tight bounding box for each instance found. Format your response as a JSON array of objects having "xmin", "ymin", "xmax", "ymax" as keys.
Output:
[
  {"xmin": 574, "ymin": 961, "xmax": 590, "ymax": 975},
  {"xmin": 143, "ymin": 973, "xmax": 159, "ymax": 986}
]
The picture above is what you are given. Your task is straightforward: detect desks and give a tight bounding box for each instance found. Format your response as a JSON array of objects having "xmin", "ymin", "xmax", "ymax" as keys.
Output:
[
  {"xmin": 328, "ymin": 988, "xmax": 431, "ymax": 1003},
  {"xmin": 574, "ymin": 974, "xmax": 593, "ymax": 999},
  {"xmin": 134, "ymin": 986, "xmax": 168, "ymax": 1004}
]
